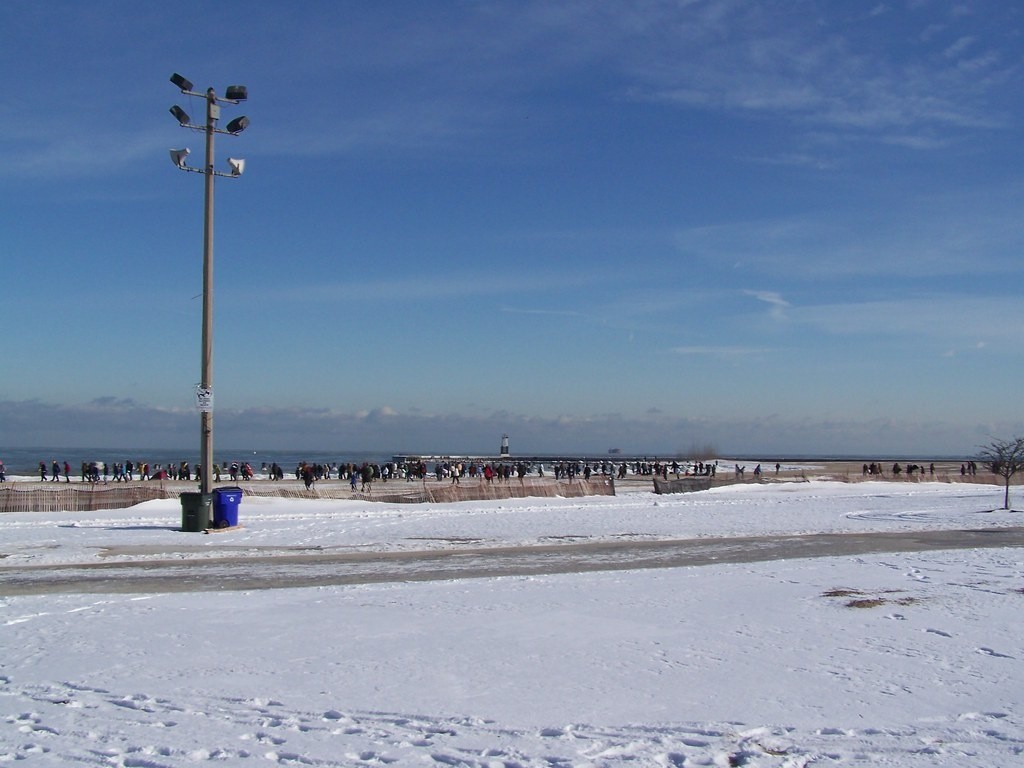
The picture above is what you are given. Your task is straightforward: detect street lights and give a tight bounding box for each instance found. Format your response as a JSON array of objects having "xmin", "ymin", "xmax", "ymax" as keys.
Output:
[{"xmin": 171, "ymin": 72, "xmax": 251, "ymax": 494}]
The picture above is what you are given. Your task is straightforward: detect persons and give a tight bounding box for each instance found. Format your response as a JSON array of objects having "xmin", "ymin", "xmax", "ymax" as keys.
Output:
[
  {"xmin": 0, "ymin": 460, "xmax": 6, "ymax": 483},
  {"xmin": 862, "ymin": 462, "xmax": 936, "ymax": 475},
  {"xmin": 775, "ymin": 462, "xmax": 780, "ymax": 475},
  {"xmin": 754, "ymin": 464, "xmax": 761, "ymax": 477},
  {"xmin": 36, "ymin": 459, "xmax": 746, "ymax": 493},
  {"xmin": 960, "ymin": 461, "xmax": 977, "ymax": 476}
]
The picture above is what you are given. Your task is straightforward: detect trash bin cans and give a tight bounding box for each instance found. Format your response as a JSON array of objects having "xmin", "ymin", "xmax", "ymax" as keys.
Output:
[
  {"xmin": 212, "ymin": 486, "xmax": 244, "ymax": 530},
  {"xmin": 179, "ymin": 492, "xmax": 213, "ymax": 532}
]
